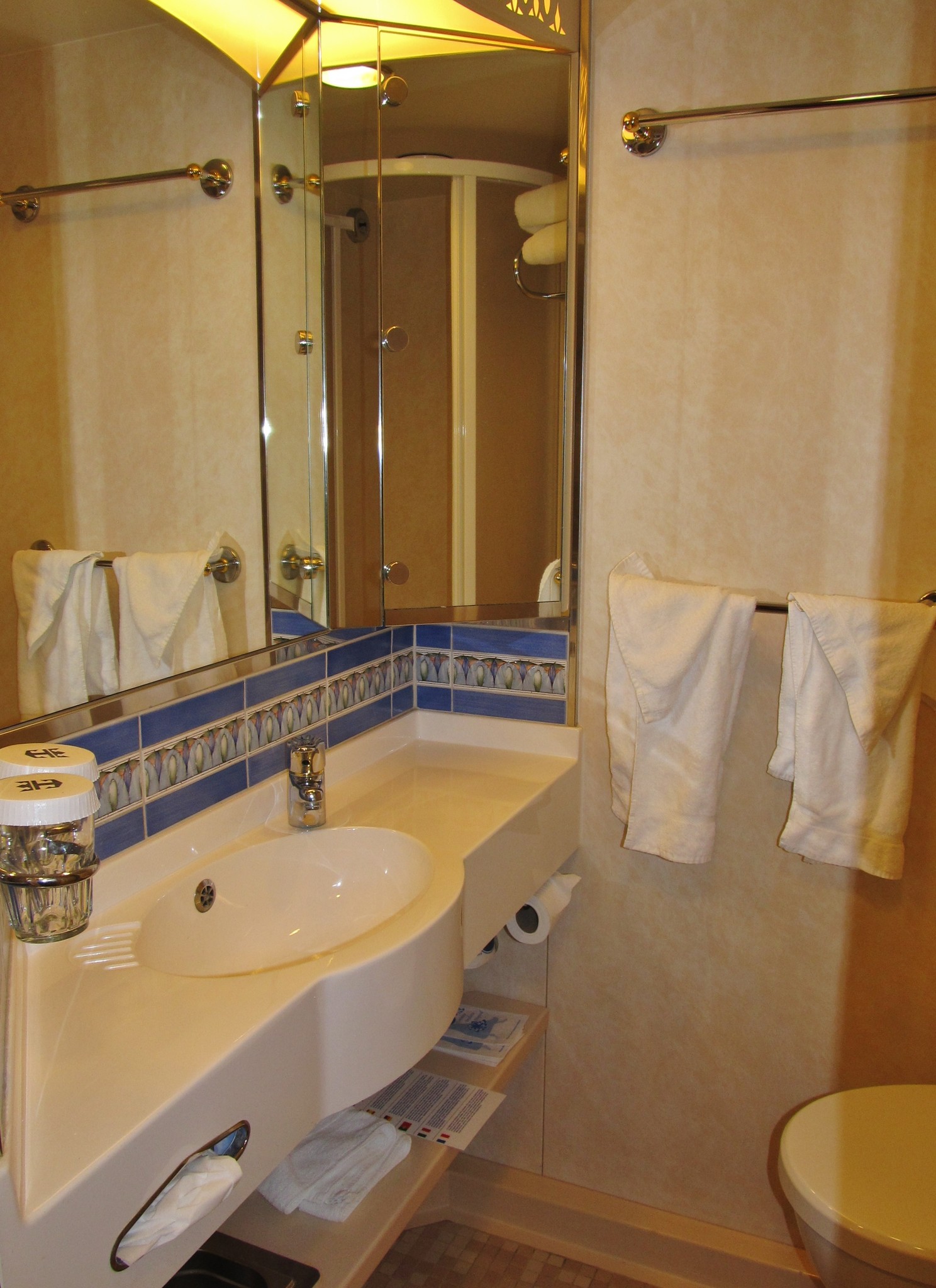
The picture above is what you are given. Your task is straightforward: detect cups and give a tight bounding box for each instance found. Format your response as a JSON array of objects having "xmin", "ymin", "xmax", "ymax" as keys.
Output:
[{"xmin": 0, "ymin": 817, "xmax": 96, "ymax": 945}]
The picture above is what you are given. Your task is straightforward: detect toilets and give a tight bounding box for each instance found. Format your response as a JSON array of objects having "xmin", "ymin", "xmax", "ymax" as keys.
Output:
[{"xmin": 774, "ymin": 1083, "xmax": 935, "ymax": 1288}]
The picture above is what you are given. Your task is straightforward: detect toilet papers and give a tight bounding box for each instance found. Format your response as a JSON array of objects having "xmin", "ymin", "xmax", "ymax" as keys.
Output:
[{"xmin": 508, "ymin": 868, "xmax": 578, "ymax": 946}]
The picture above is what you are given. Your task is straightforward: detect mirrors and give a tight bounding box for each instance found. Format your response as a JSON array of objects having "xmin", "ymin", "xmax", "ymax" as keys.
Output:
[{"xmin": 0, "ymin": 1, "xmax": 590, "ymax": 736}]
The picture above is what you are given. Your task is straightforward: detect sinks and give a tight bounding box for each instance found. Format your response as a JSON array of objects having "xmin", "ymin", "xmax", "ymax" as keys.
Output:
[{"xmin": 132, "ymin": 822, "xmax": 432, "ymax": 983}]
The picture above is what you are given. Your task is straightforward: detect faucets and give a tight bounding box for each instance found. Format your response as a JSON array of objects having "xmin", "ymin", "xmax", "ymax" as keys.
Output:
[{"xmin": 287, "ymin": 736, "xmax": 328, "ymax": 830}]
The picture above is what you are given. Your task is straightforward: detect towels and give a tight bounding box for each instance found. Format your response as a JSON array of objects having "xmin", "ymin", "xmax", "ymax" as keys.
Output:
[
  {"xmin": 537, "ymin": 559, "xmax": 562, "ymax": 603},
  {"xmin": 256, "ymin": 1102, "xmax": 413, "ymax": 1226},
  {"xmin": 109, "ymin": 552, "xmax": 238, "ymax": 718},
  {"xmin": 10, "ymin": 545, "xmax": 119, "ymax": 740},
  {"xmin": 766, "ymin": 592, "xmax": 934, "ymax": 883},
  {"xmin": 510, "ymin": 179, "xmax": 568, "ymax": 266},
  {"xmin": 603, "ymin": 572, "xmax": 761, "ymax": 868},
  {"xmin": 114, "ymin": 1145, "xmax": 243, "ymax": 1267}
]
